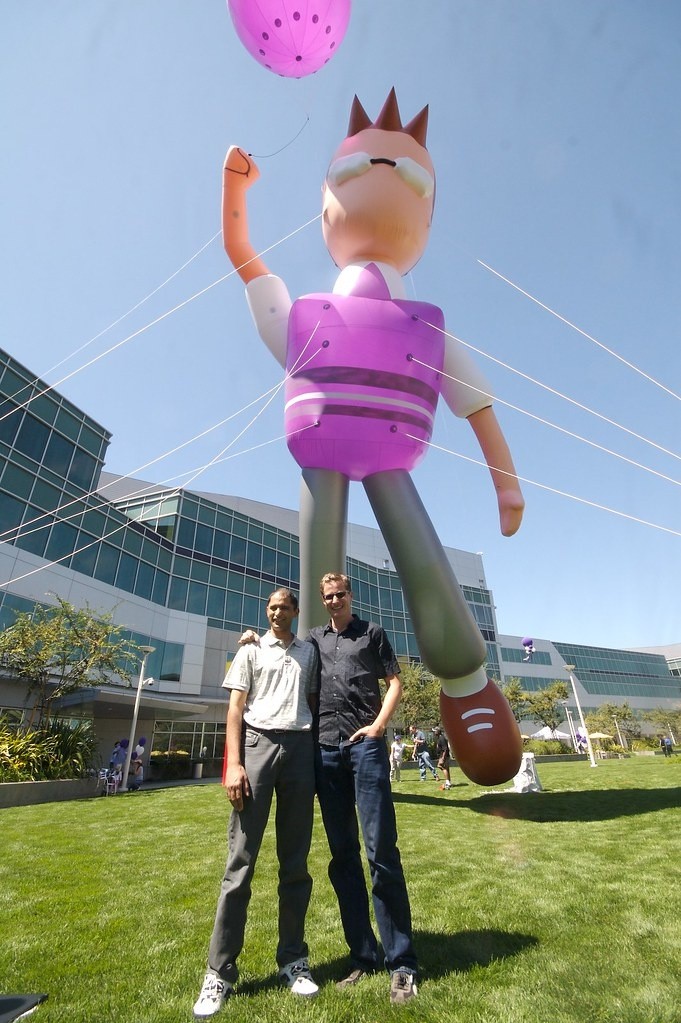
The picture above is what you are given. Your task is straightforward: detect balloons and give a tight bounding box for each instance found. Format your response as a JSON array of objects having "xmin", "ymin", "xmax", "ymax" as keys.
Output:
[
  {"xmin": 120, "ymin": 740, "xmax": 129, "ymax": 748},
  {"xmin": 576, "ymin": 727, "xmax": 588, "ymax": 747},
  {"xmin": 522, "ymin": 636, "xmax": 536, "ymax": 653},
  {"xmin": 132, "ymin": 737, "xmax": 146, "ymax": 760}
]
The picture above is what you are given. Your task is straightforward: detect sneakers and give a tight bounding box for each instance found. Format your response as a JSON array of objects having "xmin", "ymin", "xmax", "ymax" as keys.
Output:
[
  {"xmin": 390, "ymin": 970, "xmax": 418, "ymax": 1005},
  {"xmin": 336, "ymin": 961, "xmax": 374, "ymax": 988},
  {"xmin": 193, "ymin": 972, "xmax": 233, "ymax": 1017},
  {"xmin": 278, "ymin": 956, "xmax": 320, "ymax": 996}
]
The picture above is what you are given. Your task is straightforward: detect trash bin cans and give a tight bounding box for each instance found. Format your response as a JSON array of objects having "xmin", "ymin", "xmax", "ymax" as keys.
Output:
[{"xmin": 192, "ymin": 763, "xmax": 203, "ymax": 779}]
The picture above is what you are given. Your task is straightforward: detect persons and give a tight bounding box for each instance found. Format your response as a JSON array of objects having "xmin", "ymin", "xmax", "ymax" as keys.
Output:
[
  {"xmin": 129, "ymin": 759, "xmax": 143, "ymax": 792},
  {"xmin": 390, "ymin": 731, "xmax": 415, "ymax": 781},
  {"xmin": 411, "ymin": 724, "xmax": 453, "ymax": 790},
  {"xmin": 659, "ymin": 736, "xmax": 679, "ymax": 758},
  {"xmin": 194, "ymin": 589, "xmax": 322, "ymax": 1017},
  {"xmin": 108, "ymin": 740, "xmax": 127, "ymax": 770},
  {"xmin": 238, "ymin": 572, "xmax": 422, "ymax": 1007}
]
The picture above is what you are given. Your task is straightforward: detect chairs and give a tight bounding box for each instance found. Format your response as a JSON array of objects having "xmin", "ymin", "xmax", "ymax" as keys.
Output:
[{"xmin": 96, "ymin": 768, "xmax": 122, "ymax": 797}]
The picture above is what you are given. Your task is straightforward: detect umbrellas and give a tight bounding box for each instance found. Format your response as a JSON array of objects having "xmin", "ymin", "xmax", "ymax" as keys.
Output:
[
  {"xmin": 531, "ymin": 726, "xmax": 573, "ymax": 740},
  {"xmin": 588, "ymin": 731, "xmax": 612, "ymax": 745}
]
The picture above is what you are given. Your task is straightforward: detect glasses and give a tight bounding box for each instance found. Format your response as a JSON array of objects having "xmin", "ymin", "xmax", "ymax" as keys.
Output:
[{"xmin": 322, "ymin": 591, "xmax": 350, "ymax": 598}]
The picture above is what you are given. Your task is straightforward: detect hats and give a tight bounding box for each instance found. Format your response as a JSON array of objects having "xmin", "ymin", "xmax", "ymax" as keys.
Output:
[
  {"xmin": 395, "ymin": 735, "xmax": 403, "ymax": 741},
  {"xmin": 134, "ymin": 759, "xmax": 142, "ymax": 763},
  {"xmin": 432, "ymin": 727, "xmax": 442, "ymax": 732}
]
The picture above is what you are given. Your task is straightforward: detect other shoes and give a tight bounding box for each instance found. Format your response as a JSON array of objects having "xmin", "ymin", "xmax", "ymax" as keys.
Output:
[
  {"xmin": 436, "ymin": 775, "xmax": 440, "ymax": 781},
  {"xmin": 129, "ymin": 786, "xmax": 143, "ymax": 791},
  {"xmin": 444, "ymin": 784, "xmax": 452, "ymax": 788}
]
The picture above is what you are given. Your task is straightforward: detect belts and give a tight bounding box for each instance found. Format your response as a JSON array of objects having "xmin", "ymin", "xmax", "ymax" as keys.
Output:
[{"xmin": 242, "ymin": 722, "xmax": 312, "ymax": 735}]
